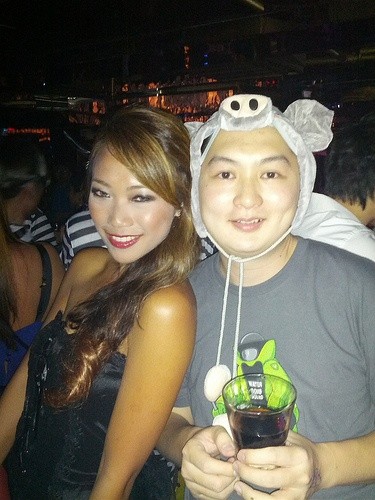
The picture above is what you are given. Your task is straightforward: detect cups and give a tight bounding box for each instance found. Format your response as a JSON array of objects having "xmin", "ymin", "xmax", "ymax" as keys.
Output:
[{"xmin": 222, "ymin": 373, "xmax": 297, "ymax": 469}]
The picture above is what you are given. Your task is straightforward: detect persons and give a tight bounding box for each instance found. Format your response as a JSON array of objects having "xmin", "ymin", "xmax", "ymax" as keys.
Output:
[
  {"xmin": 0, "ymin": 103, "xmax": 197, "ymax": 500},
  {"xmin": 0, "ymin": 176, "xmax": 67, "ymax": 499},
  {"xmin": 157, "ymin": 94, "xmax": 374, "ymax": 500},
  {"xmin": 291, "ymin": 116, "xmax": 374, "ymax": 261},
  {"xmin": 0, "ymin": 126, "xmax": 220, "ymax": 271}
]
